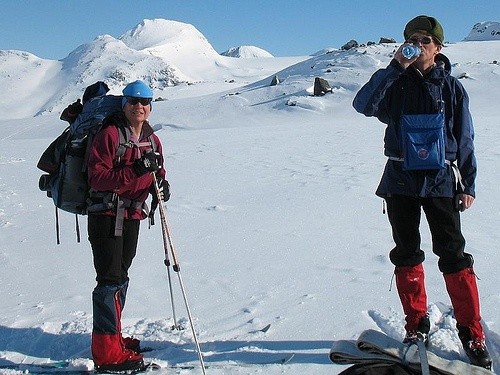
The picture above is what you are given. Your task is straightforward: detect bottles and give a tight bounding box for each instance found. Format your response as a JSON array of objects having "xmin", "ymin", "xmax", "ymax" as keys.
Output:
[{"xmin": 402, "ymin": 45, "xmax": 422, "ymax": 59}]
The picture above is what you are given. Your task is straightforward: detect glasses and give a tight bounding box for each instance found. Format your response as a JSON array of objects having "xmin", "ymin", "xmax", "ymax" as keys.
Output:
[
  {"xmin": 126, "ymin": 96, "xmax": 152, "ymax": 106},
  {"xmin": 408, "ymin": 37, "xmax": 432, "ymax": 44}
]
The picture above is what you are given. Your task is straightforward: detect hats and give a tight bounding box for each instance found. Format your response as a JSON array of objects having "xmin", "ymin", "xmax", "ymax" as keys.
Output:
[
  {"xmin": 122, "ymin": 80, "xmax": 153, "ymax": 112},
  {"xmin": 403, "ymin": 15, "xmax": 444, "ymax": 44}
]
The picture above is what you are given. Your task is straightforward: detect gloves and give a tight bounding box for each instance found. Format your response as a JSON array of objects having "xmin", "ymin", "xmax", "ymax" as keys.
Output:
[
  {"xmin": 132, "ymin": 152, "xmax": 163, "ymax": 177},
  {"xmin": 160, "ymin": 179, "xmax": 170, "ymax": 202}
]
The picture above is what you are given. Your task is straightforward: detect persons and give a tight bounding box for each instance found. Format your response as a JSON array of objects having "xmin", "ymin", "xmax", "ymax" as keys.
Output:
[
  {"xmin": 87, "ymin": 80, "xmax": 170, "ymax": 374},
  {"xmin": 353, "ymin": 15, "xmax": 493, "ymax": 372}
]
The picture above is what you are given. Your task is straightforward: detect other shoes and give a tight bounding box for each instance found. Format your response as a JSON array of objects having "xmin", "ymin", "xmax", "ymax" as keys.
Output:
[
  {"xmin": 402, "ymin": 312, "xmax": 430, "ymax": 349},
  {"xmin": 124, "ymin": 337, "xmax": 141, "ymax": 354},
  {"xmin": 95, "ymin": 354, "xmax": 144, "ymax": 373},
  {"xmin": 456, "ymin": 322, "xmax": 492, "ymax": 369}
]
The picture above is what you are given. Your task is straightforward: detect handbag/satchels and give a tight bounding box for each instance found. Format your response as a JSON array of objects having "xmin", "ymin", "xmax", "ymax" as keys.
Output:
[{"xmin": 400, "ymin": 114, "xmax": 446, "ymax": 171}]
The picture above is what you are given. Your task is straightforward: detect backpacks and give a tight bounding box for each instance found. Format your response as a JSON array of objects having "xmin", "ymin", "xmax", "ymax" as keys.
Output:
[{"xmin": 37, "ymin": 80, "xmax": 157, "ymax": 215}]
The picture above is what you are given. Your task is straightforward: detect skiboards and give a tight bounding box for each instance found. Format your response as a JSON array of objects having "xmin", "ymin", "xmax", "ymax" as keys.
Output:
[
  {"xmin": 428, "ymin": 307, "xmax": 494, "ymax": 371},
  {"xmin": 0, "ymin": 321, "xmax": 272, "ymax": 374}
]
